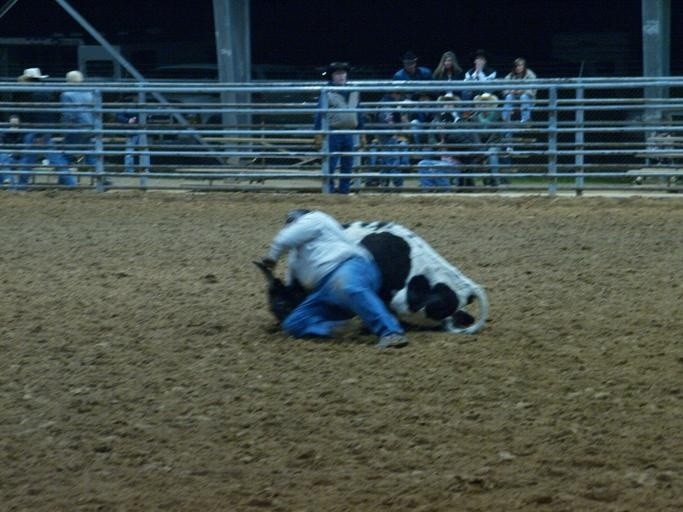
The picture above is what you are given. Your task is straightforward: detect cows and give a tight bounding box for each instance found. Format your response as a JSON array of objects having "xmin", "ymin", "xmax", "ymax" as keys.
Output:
[{"xmin": 251, "ymin": 219, "xmax": 490, "ymax": 336}]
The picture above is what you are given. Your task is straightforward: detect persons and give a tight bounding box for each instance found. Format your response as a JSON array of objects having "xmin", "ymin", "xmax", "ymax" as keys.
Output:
[
  {"xmin": 314, "ymin": 61, "xmax": 365, "ymax": 196},
  {"xmin": 371, "ymin": 52, "xmax": 537, "ymax": 188},
  {"xmin": 633, "ymin": 115, "xmax": 678, "ymax": 188},
  {"xmin": 8, "ymin": 67, "xmax": 75, "ymax": 187},
  {"xmin": 264, "ymin": 207, "xmax": 408, "ymax": 348},
  {"xmin": 58, "ymin": 72, "xmax": 112, "ymax": 193}
]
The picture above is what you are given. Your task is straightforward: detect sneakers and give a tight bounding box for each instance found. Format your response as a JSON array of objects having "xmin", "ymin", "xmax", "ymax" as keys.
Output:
[{"xmin": 372, "ymin": 332, "xmax": 409, "ymax": 349}]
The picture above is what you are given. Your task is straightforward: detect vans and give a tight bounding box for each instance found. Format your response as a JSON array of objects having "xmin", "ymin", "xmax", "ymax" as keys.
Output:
[{"xmin": 113, "ymin": 63, "xmax": 320, "ymax": 162}]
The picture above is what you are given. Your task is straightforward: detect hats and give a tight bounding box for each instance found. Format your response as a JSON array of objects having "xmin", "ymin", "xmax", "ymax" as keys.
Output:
[
  {"xmin": 64, "ymin": 70, "xmax": 84, "ymax": 83},
  {"xmin": 17, "ymin": 66, "xmax": 49, "ymax": 80},
  {"xmin": 436, "ymin": 92, "xmax": 462, "ymax": 108},
  {"xmin": 320, "ymin": 62, "xmax": 354, "ymax": 79},
  {"xmin": 410, "ymin": 92, "xmax": 437, "ymax": 101},
  {"xmin": 473, "ymin": 93, "xmax": 499, "ymax": 108}
]
